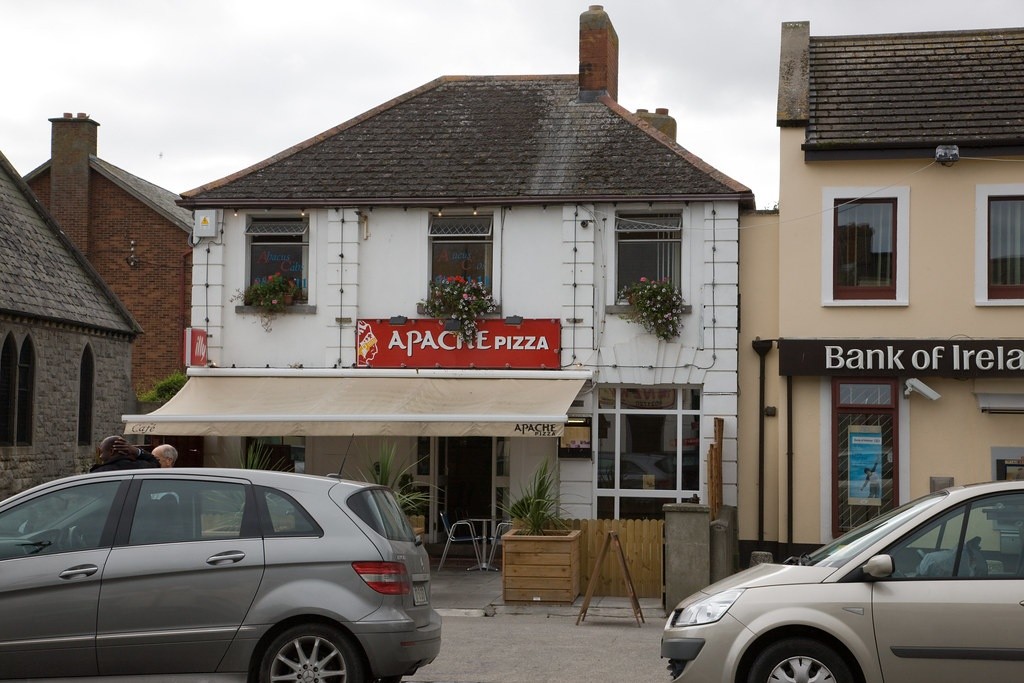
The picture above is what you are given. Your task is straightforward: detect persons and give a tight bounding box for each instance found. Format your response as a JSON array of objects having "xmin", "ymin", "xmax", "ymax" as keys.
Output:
[
  {"xmin": 860, "ymin": 458, "xmax": 882, "ymax": 498},
  {"xmin": 77, "ymin": 435, "xmax": 182, "ymax": 548}
]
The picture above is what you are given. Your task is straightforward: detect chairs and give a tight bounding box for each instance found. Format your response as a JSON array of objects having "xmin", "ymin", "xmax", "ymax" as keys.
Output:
[
  {"xmin": 438, "ymin": 509, "xmax": 483, "ymax": 572},
  {"xmin": 485, "ymin": 520, "xmax": 514, "ymax": 572}
]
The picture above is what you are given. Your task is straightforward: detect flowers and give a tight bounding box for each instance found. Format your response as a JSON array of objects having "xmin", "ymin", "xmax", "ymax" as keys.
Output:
[
  {"xmin": 416, "ymin": 274, "xmax": 497, "ymax": 343},
  {"xmin": 230, "ymin": 272, "xmax": 298, "ymax": 332},
  {"xmin": 618, "ymin": 277, "xmax": 686, "ymax": 343}
]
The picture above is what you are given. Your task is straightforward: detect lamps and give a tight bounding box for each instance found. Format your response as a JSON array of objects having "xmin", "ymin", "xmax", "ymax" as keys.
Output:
[
  {"xmin": 389, "ymin": 316, "xmax": 407, "ymax": 326},
  {"xmin": 935, "ymin": 144, "xmax": 959, "ymax": 167},
  {"xmin": 444, "ymin": 318, "xmax": 461, "ymax": 330},
  {"xmin": 504, "ymin": 315, "xmax": 523, "ymax": 326}
]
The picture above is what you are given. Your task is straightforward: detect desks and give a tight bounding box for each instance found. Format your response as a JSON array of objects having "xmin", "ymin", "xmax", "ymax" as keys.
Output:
[{"xmin": 465, "ymin": 517, "xmax": 499, "ymax": 571}]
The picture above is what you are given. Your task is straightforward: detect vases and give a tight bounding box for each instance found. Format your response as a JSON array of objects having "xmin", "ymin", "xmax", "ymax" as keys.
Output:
[
  {"xmin": 628, "ymin": 297, "xmax": 633, "ymax": 304},
  {"xmin": 285, "ymin": 293, "xmax": 294, "ymax": 306},
  {"xmin": 333, "ymin": 438, "xmax": 447, "ymax": 548}
]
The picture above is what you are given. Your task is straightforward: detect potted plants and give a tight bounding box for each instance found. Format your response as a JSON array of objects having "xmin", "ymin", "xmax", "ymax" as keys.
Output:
[{"xmin": 495, "ymin": 456, "xmax": 583, "ymax": 605}]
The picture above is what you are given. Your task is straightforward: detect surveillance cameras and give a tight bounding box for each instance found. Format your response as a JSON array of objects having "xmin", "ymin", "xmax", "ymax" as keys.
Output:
[{"xmin": 904, "ymin": 378, "xmax": 941, "ymax": 401}]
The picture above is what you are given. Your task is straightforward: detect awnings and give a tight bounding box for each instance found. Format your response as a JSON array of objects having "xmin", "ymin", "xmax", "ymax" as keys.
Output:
[{"xmin": 118, "ymin": 367, "xmax": 594, "ymax": 437}]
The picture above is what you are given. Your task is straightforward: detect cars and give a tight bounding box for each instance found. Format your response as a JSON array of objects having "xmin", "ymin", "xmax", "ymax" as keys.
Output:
[
  {"xmin": 657, "ymin": 481, "xmax": 1023, "ymax": 682},
  {"xmin": 0, "ymin": 465, "xmax": 440, "ymax": 682}
]
